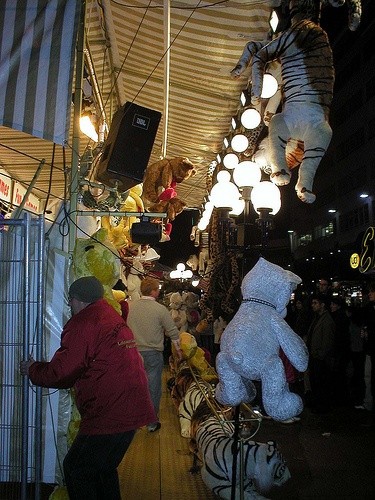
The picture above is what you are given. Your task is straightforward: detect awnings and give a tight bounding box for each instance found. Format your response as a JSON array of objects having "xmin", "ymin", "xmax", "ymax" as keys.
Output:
[{"xmin": 110, "ymin": 0, "xmax": 280, "ymax": 210}]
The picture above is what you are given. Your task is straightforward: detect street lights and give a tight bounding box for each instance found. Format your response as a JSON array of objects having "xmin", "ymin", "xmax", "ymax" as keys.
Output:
[{"xmin": 211, "ymin": 162, "xmax": 282, "ymax": 286}]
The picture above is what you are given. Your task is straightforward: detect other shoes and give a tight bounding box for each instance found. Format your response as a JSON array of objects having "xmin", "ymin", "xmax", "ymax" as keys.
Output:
[
  {"xmin": 148, "ymin": 422, "xmax": 161, "ymax": 433},
  {"xmin": 354, "ymin": 405, "xmax": 365, "ymax": 410}
]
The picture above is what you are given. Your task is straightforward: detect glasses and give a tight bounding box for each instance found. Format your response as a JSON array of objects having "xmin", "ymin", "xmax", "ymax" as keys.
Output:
[{"xmin": 68, "ymin": 296, "xmax": 75, "ymax": 301}]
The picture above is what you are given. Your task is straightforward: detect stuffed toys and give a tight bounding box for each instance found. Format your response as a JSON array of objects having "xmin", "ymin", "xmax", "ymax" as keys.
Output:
[
  {"xmin": 230, "ymin": 15, "xmax": 334, "ymax": 204},
  {"xmin": 70, "ymin": 162, "xmax": 314, "ymax": 500},
  {"xmin": 142, "ymin": 157, "xmax": 197, "ymax": 203},
  {"xmin": 215, "ymin": 258, "xmax": 310, "ymax": 420}
]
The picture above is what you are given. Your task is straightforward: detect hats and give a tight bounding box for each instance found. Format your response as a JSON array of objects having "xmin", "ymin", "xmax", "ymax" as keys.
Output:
[{"xmin": 69, "ymin": 276, "xmax": 104, "ymax": 302}]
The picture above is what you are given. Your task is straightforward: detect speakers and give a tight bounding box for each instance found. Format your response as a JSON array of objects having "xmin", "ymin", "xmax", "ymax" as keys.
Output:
[
  {"xmin": 93, "ymin": 101, "xmax": 161, "ymax": 193},
  {"xmin": 131, "ymin": 222, "xmax": 162, "ymax": 244}
]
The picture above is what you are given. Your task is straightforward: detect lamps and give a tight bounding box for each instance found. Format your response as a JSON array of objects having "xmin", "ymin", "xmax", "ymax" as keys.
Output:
[
  {"xmin": 80, "ymin": 110, "xmax": 99, "ymax": 142},
  {"xmin": 196, "ymin": 4, "xmax": 295, "ymax": 256},
  {"xmin": 168, "ymin": 261, "xmax": 194, "ymax": 282},
  {"xmin": 126, "ymin": 216, "xmax": 165, "ymax": 247}
]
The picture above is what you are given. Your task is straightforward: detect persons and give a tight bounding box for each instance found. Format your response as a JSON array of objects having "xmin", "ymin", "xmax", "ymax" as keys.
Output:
[
  {"xmin": 271, "ymin": 278, "xmax": 375, "ymax": 436},
  {"xmin": 126, "ymin": 277, "xmax": 181, "ymax": 432},
  {"xmin": 19, "ymin": 276, "xmax": 157, "ymax": 500}
]
